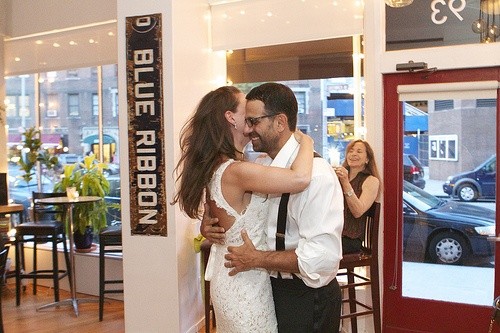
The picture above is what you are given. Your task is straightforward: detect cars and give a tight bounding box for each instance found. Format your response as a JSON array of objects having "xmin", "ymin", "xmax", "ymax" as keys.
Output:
[
  {"xmin": 7, "ymin": 143, "xmax": 122, "ymax": 246},
  {"xmin": 442, "ymin": 154, "xmax": 496, "ymax": 203},
  {"xmin": 402, "ymin": 180, "xmax": 497, "ymax": 267}
]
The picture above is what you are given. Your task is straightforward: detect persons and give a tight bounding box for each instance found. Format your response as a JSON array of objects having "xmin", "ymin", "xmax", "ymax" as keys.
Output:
[
  {"xmin": 201, "ymin": 83, "xmax": 344, "ymax": 333},
  {"xmin": 170, "ymin": 85, "xmax": 314, "ymax": 333},
  {"xmin": 332, "ymin": 139, "xmax": 382, "ymax": 255}
]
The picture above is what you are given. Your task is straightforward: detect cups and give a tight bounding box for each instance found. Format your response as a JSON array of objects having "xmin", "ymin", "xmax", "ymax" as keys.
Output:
[{"xmin": 66, "ymin": 187, "xmax": 79, "ymax": 200}]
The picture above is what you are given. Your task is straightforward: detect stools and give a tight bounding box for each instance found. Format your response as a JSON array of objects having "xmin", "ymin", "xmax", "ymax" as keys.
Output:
[
  {"xmin": 14, "ymin": 191, "xmax": 73, "ymax": 309},
  {"xmin": 335, "ymin": 201, "xmax": 381, "ymax": 333},
  {"xmin": 99, "ymin": 225, "xmax": 123, "ymax": 321},
  {"xmin": 200, "ymin": 238, "xmax": 216, "ymax": 333}
]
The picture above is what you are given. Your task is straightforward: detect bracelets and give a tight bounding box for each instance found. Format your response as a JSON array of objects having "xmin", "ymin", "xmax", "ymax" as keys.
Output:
[{"xmin": 344, "ymin": 191, "xmax": 352, "ymax": 196}]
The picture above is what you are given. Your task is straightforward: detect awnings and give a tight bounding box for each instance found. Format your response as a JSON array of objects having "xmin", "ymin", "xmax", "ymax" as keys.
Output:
[
  {"xmin": 8, "ymin": 134, "xmax": 61, "ymax": 143},
  {"xmin": 83, "ymin": 134, "xmax": 114, "ymax": 144}
]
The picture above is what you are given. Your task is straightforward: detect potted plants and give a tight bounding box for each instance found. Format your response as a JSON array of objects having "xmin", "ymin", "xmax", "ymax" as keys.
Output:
[
  {"xmin": 7, "ymin": 126, "xmax": 68, "ymax": 224},
  {"xmin": 52, "ymin": 150, "xmax": 112, "ymax": 248}
]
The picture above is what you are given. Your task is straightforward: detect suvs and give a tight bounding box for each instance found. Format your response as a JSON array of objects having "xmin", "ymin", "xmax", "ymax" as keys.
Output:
[{"xmin": 402, "ymin": 153, "xmax": 426, "ymax": 189}]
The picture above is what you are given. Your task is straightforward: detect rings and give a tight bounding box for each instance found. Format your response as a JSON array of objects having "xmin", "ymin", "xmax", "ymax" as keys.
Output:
[
  {"xmin": 342, "ymin": 172, "xmax": 343, "ymax": 175},
  {"xmin": 230, "ymin": 261, "xmax": 232, "ymax": 266}
]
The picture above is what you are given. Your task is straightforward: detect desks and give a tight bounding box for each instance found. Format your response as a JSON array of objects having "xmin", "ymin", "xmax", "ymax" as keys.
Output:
[{"xmin": 34, "ymin": 195, "xmax": 111, "ymax": 318}]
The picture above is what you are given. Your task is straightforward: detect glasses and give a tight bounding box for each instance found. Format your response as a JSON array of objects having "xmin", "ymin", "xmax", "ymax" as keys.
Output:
[{"xmin": 245, "ymin": 113, "xmax": 280, "ymax": 127}]
